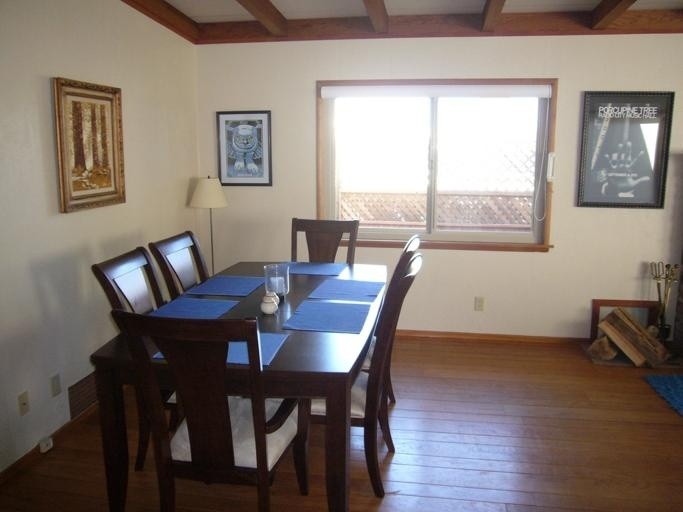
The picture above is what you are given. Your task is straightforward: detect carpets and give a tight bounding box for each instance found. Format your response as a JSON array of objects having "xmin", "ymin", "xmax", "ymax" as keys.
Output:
[{"xmin": 641, "ymin": 368, "xmax": 683, "ymax": 413}]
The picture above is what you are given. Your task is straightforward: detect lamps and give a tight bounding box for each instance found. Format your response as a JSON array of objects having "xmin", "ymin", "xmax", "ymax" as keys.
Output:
[{"xmin": 188, "ymin": 175, "xmax": 229, "ymax": 275}]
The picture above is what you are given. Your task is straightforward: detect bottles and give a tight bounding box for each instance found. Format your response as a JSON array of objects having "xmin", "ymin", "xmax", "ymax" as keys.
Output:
[
  {"xmin": 264, "ymin": 291, "xmax": 279, "ymax": 305},
  {"xmin": 260, "ymin": 296, "xmax": 278, "ymax": 314}
]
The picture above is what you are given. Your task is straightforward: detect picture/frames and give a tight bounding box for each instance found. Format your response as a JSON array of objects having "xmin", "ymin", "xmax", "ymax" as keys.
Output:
[
  {"xmin": 591, "ymin": 298, "xmax": 661, "ymax": 347},
  {"xmin": 577, "ymin": 91, "xmax": 675, "ymax": 208},
  {"xmin": 52, "ymin": 73, "xmax": 126, "ymax": 213},
  {"xmin": 214, "ymin": 110, "xmax": 273, "ymax": 186}
]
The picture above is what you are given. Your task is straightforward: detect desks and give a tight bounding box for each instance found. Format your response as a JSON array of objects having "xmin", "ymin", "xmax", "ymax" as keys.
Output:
[{"xmin": 92, "ymin": 261, "xmax": 388, "ymax": 512}]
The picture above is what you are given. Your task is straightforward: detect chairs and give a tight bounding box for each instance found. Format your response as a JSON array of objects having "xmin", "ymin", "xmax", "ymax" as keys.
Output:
[
  {"xmin": 310, "ymin": 252, "xmax": 423, "ymax": 495},
  {"xmin": 91, "ymin": 244, "xmax": 165, "ymax": 321},
  {"xmin": 147, "ymin": 231, "xmax": 210, "ymax": 295},
  {"xmin": 291, "ymin": 217, "xmax": 359, "ymax": 265},
  {"xmin": 111, "ymin": 309, "xmax": 311, "ymax": 512},
  {"xmin": 360, "ymin": 234, "xmax": 424, "ymax": 403}
]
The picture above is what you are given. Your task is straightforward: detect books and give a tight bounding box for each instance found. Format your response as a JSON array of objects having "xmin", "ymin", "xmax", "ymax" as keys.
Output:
[{"xmin": 596, "ymin": 307, "xmax": 662, "ymax": 367}]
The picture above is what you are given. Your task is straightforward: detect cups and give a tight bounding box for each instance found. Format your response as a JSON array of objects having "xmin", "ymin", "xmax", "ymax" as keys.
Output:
[{"xmin": 262, "ymin": 263, "xmax": 290, "ymax": 297}]
